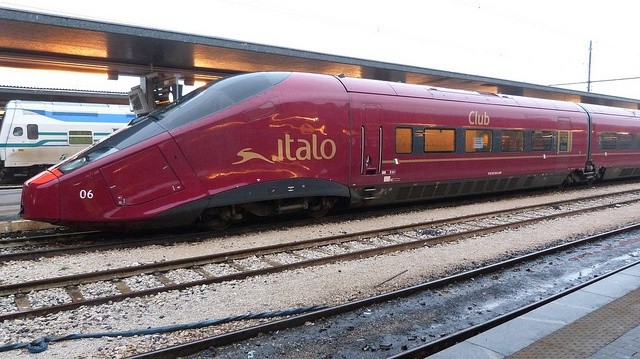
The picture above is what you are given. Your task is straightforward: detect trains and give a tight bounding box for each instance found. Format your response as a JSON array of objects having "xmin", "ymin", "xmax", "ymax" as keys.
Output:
[
  {"xmin": 0, "ymin": 97, "xmax": 137, "ymax": 180},
  {"xmin": 17, "ymin": 71, "xmax": 640, "ymax": 231}
]
